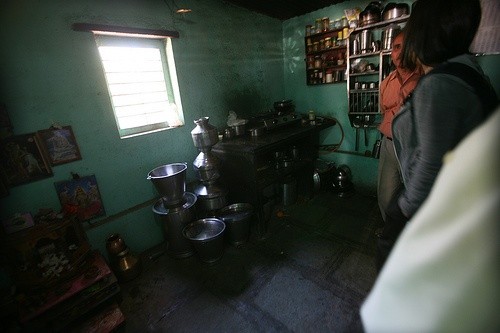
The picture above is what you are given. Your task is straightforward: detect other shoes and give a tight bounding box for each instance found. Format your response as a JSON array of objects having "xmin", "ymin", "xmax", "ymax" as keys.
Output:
[{"xmin": 375, "ymin": 228, "xmax": 399, "ymax": 239}]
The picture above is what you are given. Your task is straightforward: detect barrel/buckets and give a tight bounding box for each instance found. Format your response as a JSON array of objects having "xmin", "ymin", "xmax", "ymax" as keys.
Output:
[
  {"xmin": 146, "ymin": 161, "xmax": 188, "ymax": 206},
  {"xmin": 152, "ymin": 187, "xmax": 203, "ymax": 259},
  {"xmin": 183, "ymin": 217, "xmax": 227, "ymax": 263},
  {"xmin": 216, "ymin": 202, "xmax": 254, "ymax": 243},
  {"xmin": 279, "ymin": 148, "xmax": 301, "ymax": 206}
]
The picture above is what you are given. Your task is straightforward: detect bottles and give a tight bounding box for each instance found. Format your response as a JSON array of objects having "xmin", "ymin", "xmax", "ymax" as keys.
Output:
[
  {"xmin": 193, "ymin": 147, "xmax": 226, "ymax": 185},
  {"xmin": 193, "ymin": 185, "xmax": 229, "ymax": 216},
  {"xmin": 190, "ymin": 116, "xmax": 219, "ymax": 151},
  {"xmin": 310, "ymin": 111, "xmax": 315, "ymax": 121}
]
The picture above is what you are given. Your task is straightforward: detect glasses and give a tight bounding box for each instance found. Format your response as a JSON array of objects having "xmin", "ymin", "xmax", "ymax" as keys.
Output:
[{"xmin": 393, "ymin": 44, "xmax": 403, "ymax": 52}]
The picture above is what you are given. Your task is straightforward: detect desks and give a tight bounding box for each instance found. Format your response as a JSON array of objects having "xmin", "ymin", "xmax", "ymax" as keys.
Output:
[{"xmin": 0, "ymin": 249, "xmax": 119, "ymax": 333}]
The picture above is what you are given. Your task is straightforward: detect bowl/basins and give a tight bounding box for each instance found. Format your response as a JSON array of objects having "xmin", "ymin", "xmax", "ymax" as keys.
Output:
[{"xmin": 219, "ymin": 119, "xmax": 261, "ymax": 140}]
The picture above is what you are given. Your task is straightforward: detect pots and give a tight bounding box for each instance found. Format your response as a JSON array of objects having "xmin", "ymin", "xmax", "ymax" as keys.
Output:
[{"xmin": 274, "ymin": 101, "xmax": 292, "ymax": 111}]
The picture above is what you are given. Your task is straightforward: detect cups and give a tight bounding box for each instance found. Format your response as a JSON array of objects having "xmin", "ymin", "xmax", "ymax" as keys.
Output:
[
  {"xmin": 352, "ymin": 23, "xmax": 401, "ymax": 55},
  {"xmin": 354, "ymin": 82, "xmax": 376, "ymax": 91}
]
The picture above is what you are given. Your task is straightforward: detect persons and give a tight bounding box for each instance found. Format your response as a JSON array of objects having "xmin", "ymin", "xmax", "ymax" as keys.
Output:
[
  {"xmin": 359, "ymin": 110, "xmax": 500, "ymax": 333},
  {"xmin": 75, "ymin": 185, "xmax": 88, "ymax": 206},
  {"xmin": 384, "ymin": 0, "xmax": 498, "ymax": 256},
  {"xmin": 88, "ymin": 180, "xmax": 98, "ymax": 202},
  {"xmin": 377, "ymin": 29, "xmax": 424, "ymax": 238},
  {"xmin": 60, "ymin": 183, "xmax": 72, "ymax": 203},
  {"xmin": 12, "ymin": 144, "xmax": 40, "ymax": 173},
  {"xmin": 47, "ymin": 130, "xmax": 76, "ymax": 161}
]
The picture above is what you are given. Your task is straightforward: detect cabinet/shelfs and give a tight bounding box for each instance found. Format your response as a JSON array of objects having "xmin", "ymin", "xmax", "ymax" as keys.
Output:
[
  {"xmin": 306, "ymin": 26, "xmax": 349, "ymax": 87},
  {"xmin": 348, "ymin": 15, "xmax": 416, "ymax": 113},
  {"xmin": 196, "ymin": 118, "xmax": 339, "ymax": 238}
]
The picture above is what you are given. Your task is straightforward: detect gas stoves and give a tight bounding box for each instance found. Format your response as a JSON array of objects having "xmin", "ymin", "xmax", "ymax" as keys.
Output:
[{"xmin": 251, "ymin": 111, "xmax": 304, "ymax": 129}]
